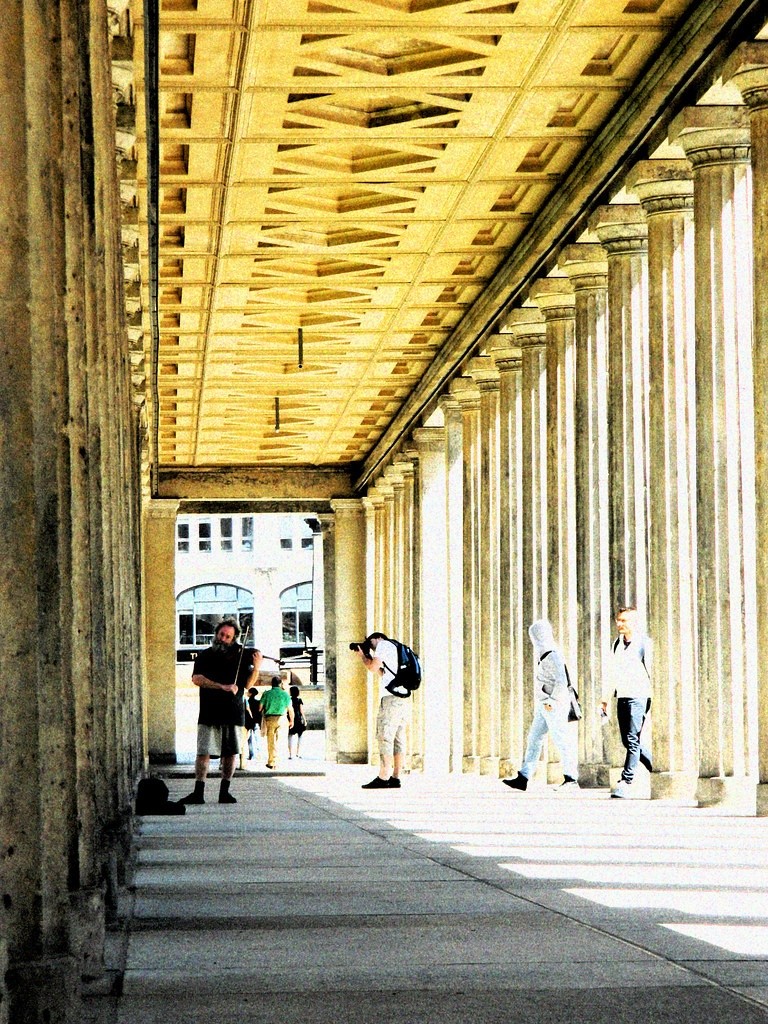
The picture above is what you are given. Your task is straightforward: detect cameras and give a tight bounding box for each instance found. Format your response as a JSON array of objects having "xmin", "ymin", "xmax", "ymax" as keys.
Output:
[{"xmin": 349, "ymin": 637, "xmax": 370, "ymax": 659}]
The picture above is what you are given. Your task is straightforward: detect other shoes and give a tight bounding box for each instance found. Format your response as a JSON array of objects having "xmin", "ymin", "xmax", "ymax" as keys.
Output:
[
  {"xmin": 248, "ymin": 754, "xmax": 254, "ymax": 759},
  {"xmin": 502, "ymin": 772, "xmax": 528, "ymax": 790},
  {"xmin": 560, "ymin": 776, "xmax": 575, "ymax": 786},
  {"xmin": 179, "ymin": 792, "xmax": 206, "ymax": 804},
  {"xmin": 218, "ymin": 791, "xmax": 237, "ymax": 804},
  {"xmin": 362, "ymin": 777, "xmax": 390, "ymax": 788},
  {"xmin": 610, "ymin": 781, "xmax": 631, "ymax": 798},
  {"xmin": 266, "ymin": 764, "xmax": 273, "ymax": 769},
  {"xmin": 389, "ymin": 777, "xmax": 401, "ymax": 788}
]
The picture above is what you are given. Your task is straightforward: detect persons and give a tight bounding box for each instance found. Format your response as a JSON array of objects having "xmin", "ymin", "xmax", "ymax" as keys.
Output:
[
  {"xmin": 287, "ymin": 686, "xmax": 307, "ymax": 759},
  {"xmin": 219, "ymin": 688, "xmax": 260, "ymax": 770},
  {"xmin": 178, "ymin": 620, "xmax": 263, "ymax": 804},
  {"xmin": 601, "ymin": 607, "xmax": 652, "ymax": 798},
  {"xmin": 354, "ymin": 632, "xmax": 411, "ymax": 788},
  {"xmin": 501, "ymin": 619, "xmax": 578, "ymax": 791},
  {"xmin": 259, "ymin": 677, "xmax": 294, "ymax": 769}
]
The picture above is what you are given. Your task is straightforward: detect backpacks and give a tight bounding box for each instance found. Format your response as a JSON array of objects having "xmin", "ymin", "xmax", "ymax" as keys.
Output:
[{"xmin": 382, "ymin": 639, "xmax": 421, "ymax": 691}]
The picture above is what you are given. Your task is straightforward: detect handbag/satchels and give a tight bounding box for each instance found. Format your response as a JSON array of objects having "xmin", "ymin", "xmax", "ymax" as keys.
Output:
[
  {"xmin": 567, "ymin": 687, "xmax": 583, "ymax": 723},
  {"xmin": 261, "ymin": 716, "xmax": 266, "ymax": 737}
]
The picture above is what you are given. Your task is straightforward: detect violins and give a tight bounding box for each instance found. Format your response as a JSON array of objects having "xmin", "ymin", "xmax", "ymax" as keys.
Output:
[{"xmin": 225, "ymin": 643, "xmax": 287, "ymax": 667}]
